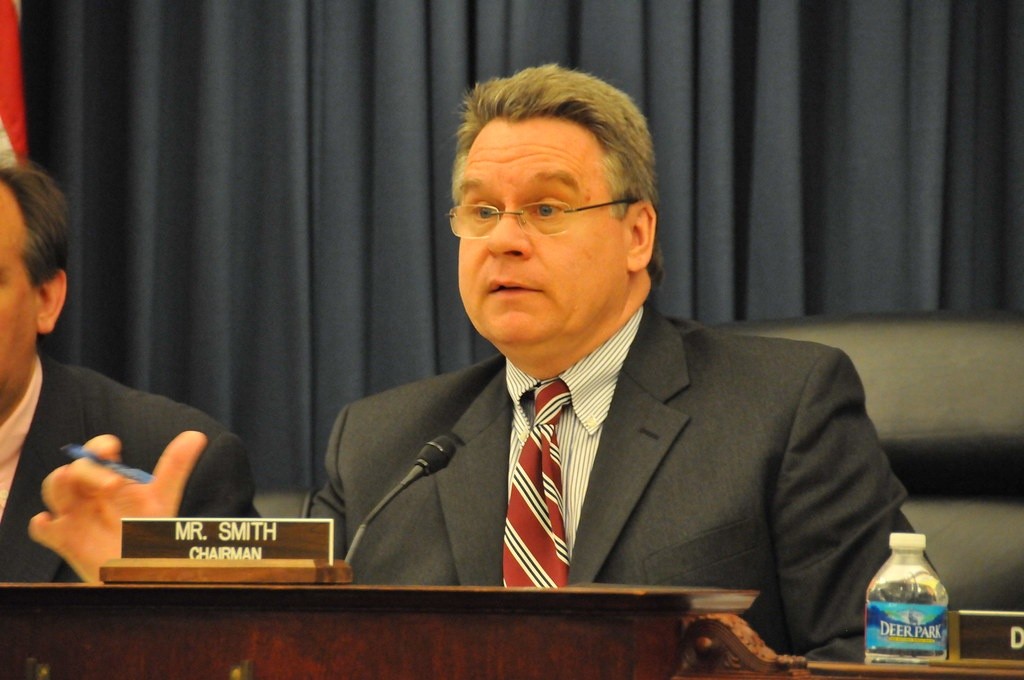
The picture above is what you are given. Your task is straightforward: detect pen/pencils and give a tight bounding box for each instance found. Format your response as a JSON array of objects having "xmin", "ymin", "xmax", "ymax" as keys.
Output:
[{"xmin": 56, "ymin": 441, "xmax": 154, "ymax": 486}]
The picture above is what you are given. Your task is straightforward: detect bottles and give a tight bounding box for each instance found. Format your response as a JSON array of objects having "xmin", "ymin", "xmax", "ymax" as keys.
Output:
[{"xmin": 866, "ymin": 533, "xmax": 947, "ymax": 664}]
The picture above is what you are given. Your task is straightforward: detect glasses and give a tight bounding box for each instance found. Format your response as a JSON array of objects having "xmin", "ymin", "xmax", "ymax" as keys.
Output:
[{"xmin": 445, "ymin": 196, "xmax": 640, "ymax": 239}]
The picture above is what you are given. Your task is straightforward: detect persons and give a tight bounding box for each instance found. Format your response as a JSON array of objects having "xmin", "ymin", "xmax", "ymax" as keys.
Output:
[
  {"xmin": 0, "ymin": 160, "xmax": 260, "ymax": 584},
  {"xmin": 26, "ymin": 63, "xmax": 944, "ymax": 665}
]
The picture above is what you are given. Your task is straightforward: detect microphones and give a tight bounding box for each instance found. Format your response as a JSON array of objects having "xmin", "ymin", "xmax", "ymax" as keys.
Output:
[{"xmin": 345, "ymin": 436, "xmax": 457, "ymax": 565}]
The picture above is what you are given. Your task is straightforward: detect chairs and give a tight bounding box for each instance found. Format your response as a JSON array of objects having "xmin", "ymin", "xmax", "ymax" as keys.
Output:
[{"xmin": 705, "ymin": 310, "xmax": 1024, "ymax": 611}]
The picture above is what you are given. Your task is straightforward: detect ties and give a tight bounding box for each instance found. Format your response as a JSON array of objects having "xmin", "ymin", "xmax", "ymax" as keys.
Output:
[{"xmin": 503, "ymin": 378, "xmax": 572, "ymax": 588}]
